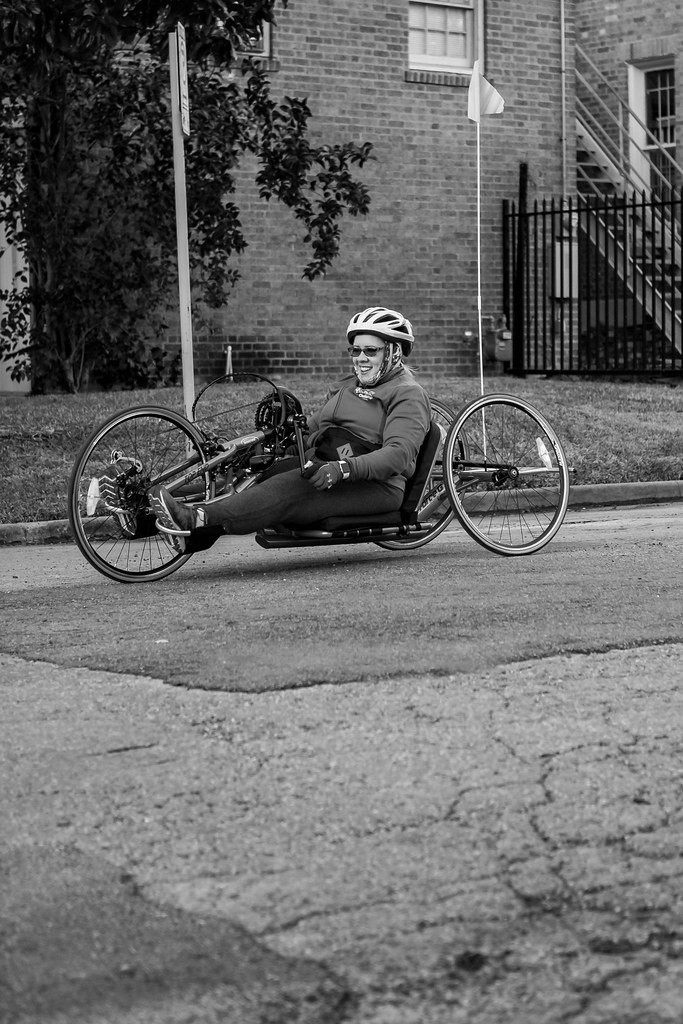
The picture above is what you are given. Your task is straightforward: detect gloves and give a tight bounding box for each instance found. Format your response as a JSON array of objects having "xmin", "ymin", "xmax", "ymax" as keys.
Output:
[{"xmin": 309, "ymin": 461, "xmax": 352, "ymax": 490}]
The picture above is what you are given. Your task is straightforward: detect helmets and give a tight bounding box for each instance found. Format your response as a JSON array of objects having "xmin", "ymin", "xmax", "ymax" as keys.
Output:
[{"xmin": 346, "ymin": 306, "xmax": 414, "ymax": 358}]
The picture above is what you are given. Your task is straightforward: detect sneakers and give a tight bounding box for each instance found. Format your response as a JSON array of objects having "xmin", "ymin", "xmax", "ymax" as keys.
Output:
[
  {"xmin": 147, "ymin": 485, "xmax": 197, "ymax": 554},
  {"xmin": 98, "ymin": 466, "xmax": 139, "ymax": 538}
]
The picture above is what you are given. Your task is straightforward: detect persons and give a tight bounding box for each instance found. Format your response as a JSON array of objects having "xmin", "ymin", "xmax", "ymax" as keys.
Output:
[{"xmin": 100, "ymin": 306, "xmax": 430, "ymax": 553}]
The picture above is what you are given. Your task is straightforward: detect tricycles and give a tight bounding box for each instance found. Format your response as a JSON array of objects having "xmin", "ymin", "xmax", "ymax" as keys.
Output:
[{"xmin": 67, "ymin": 371, "xmax": 570, "ymax": 585}]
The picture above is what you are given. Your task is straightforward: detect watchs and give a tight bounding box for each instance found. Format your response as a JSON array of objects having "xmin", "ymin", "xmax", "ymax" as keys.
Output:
[{"xmin": 337, "ymin": 461, "xmax": 350, "ymax": 479}]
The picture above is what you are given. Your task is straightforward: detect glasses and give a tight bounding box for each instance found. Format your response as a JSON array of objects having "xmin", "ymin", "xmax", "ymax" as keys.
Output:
[{"xmin": 346, "ymin": 345, "xmax": 386, "ymax": 357}]
